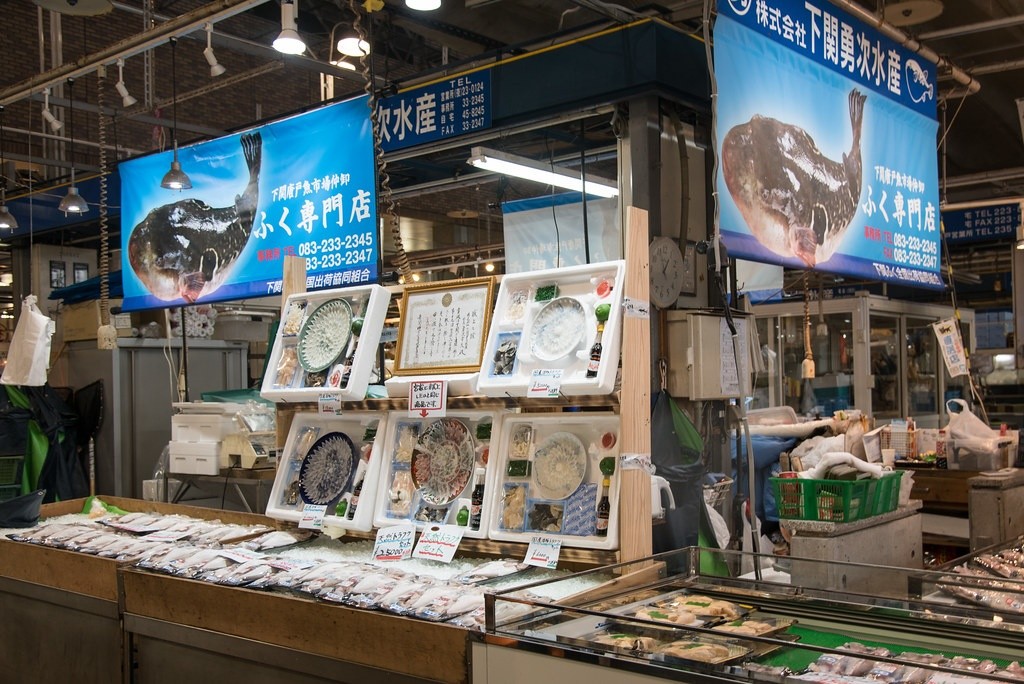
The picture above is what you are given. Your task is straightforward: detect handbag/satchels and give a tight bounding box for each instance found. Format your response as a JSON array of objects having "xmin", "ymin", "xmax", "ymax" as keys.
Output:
[{"xmin": 946, "ymin": 399, "xmax": 998, "ymax": 454}]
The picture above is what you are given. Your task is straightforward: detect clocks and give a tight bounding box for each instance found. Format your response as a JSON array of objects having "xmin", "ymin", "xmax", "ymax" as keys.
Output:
[{"xmin": 649, "ymin": 235, "xmax": 685, "ymax": 309}]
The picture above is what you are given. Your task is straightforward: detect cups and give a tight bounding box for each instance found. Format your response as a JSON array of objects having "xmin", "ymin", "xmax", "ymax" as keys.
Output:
[{"xmin": 881, "ymin": 449, "xmax": 895, "ymax": 467}]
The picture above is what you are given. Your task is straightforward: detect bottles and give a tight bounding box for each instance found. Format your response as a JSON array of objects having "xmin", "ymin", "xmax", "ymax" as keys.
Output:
[
  {"xmin": 936, "ymin": 429, "xmax": 947, "ymax": 469},
  {"xmin": 468, "ymin": 467, "xmax": 486, "ymax": 530},
  {"xmin": 346, "ymin": 474, "xmax": 364, "ymax": 521},
  {"xmin": 584, "ymin": 325, "xmax": 606, "ymax": 377},
  {"xmin": 596, "ymin": 478, "xmax": 611, "ymax": 537},
  {"xmin": 339, "ymin": 339, "xmax": 361, "ymax": 388}
]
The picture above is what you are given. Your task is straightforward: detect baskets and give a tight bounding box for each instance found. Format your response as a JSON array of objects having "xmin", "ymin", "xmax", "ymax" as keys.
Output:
[
  {"xmin": 768, "ymin": 472, "xmax": 904, "ymax": 522},
  {"xmin": 880, "ymin": 428, "xmax": 921, "ymax": 460}
]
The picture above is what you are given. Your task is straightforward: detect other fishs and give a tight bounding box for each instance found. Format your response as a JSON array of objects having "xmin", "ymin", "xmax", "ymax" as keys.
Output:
[
  {"xmin": 128, "ymin": 132, "xmax": 273, "ymax": 306},
  {"xmin": 583, "ymin": 546, "xmax": 1024, "ymax": 682},
  {"xmin": 11, "ymin": 492, "xmax": 587, "ymax": 632},
  {"xmin": 722, "ymin": 85, "xmax": 875, "ymax": 267}
]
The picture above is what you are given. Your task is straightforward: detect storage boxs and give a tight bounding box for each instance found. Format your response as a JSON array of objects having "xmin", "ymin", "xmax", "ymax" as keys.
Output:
[
  {"xmin": 0, "ymin": 415, "xmax": 30, "ymax": 503},
  {"xmin": 210, "ymin": 311, "xmax": 275, "ymax": 342},
  {"xmin": 62, "ymin": 298, "xmax": 144, "ymax": 341},
  {"xmin": 143, "ymin": 403, "xmax": 276, "ymax": 506},
  {"xmin": 699, "ymin": 426, "xmax": 1019, "ymax": 523}
]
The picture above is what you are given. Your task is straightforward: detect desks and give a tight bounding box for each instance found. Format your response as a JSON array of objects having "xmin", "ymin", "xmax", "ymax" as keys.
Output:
[{"xmin": 163, "ymin": 471, "xmax": 274, "ymax": 514}]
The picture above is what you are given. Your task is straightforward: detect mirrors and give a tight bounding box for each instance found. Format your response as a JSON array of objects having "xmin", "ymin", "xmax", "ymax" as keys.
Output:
[
  {"xmin": 50, "ymin": 260, "xmax": 67, "ymax": 288},
  {"xmin": 73, "ymin": 262, "xmax": 89, "ymax": 285}
]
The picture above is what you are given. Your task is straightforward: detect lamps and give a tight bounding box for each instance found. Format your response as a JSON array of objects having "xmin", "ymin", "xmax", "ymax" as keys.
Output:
[
  {"xmin": 0, "ymin": 0, "xmax": 620, "ymax": 284},
  {"xmin": 874, "ymin": 0, "xmax": 946, "ymax": 27}
]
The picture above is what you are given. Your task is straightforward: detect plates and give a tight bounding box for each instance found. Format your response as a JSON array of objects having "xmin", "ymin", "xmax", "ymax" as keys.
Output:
[
  {"xmin": 532, "ymin": 435, "xmax": 582, "ymax": 503},
  {"xmin": 299, "ymin": 431, "xmax": 357, "ymax": 508},
  {"xmin": 297, "ymin": 298, "xmax": 352, "ymax": 373},
  {"xmin": 529, "ymin": 299, "xmax": 585, "ymax": 362},
  {"xmin": 408, "ymin": 417, "xmax": 476, "ymax": 505}
]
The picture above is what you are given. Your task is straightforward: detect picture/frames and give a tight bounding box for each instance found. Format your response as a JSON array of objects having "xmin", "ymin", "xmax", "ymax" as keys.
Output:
[{"xmin": 393, "ymin": 276, "xmax": 497, "ymax": 376}]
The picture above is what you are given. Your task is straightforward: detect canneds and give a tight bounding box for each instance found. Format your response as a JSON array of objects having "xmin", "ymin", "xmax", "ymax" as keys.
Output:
[{"xmin": 779, "ymin": 470, "xmax": 799, "ymax": 515}]
